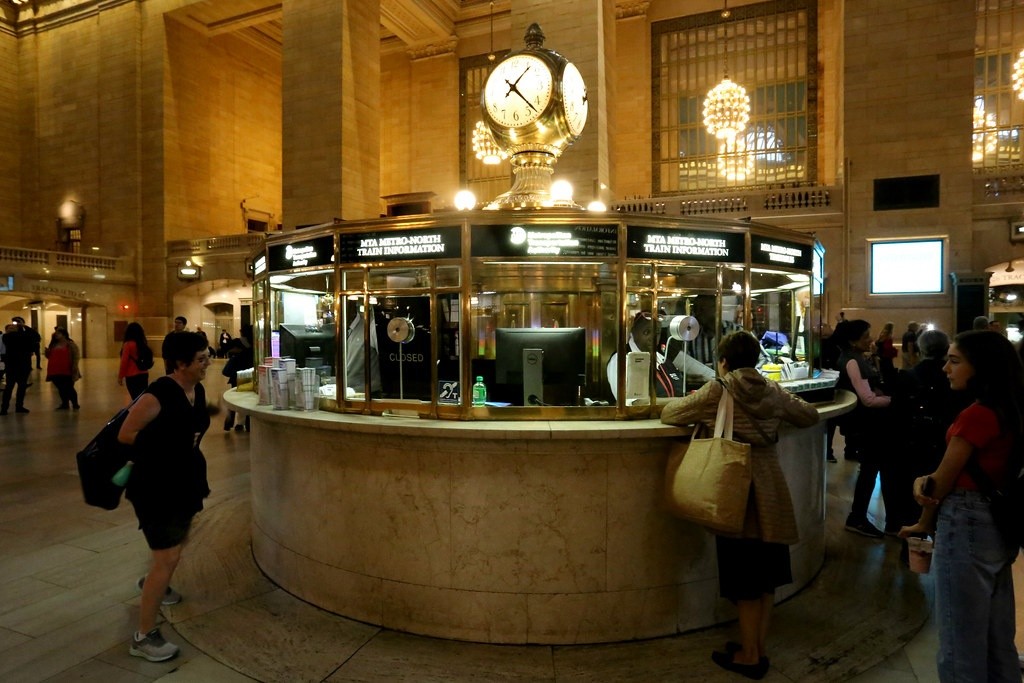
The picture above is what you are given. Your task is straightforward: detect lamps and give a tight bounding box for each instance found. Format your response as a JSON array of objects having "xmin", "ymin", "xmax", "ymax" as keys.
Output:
[
  {"xmin": 177, "ymin": 265, "xmax": 200, "ymax": 278},
  {"xmin": 701, "ymin": 0, "xmax": 750, "ymax": 138},
  {"xmin": 472, "ymin": 0, "xmax": 506, "ymax": 164}
]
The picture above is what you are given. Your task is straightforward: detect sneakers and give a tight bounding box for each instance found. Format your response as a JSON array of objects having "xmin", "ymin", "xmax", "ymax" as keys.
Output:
[
  {"xmin": 827, "ymin": 448, "xmax": 837, "ymax": 463},
  {"xmin": 129, "ymin": 628, "xmax": 179, "ymax": 662},
  {"xmin": 137, "ymin": 576, "xmax": 180, "ymax": 605},
  {"xmin": 0, "ymin": 408, "xmax": 8, "ymax": 415},
  {"xmin": 15, "ymin": 403, "xmax": 30, "ymax": 414},
  {"xmin": 844, "ymin": 450, "xmax": 860, "ymax": 460},
  {"xmin": 73, "ymin": 404, "xmax": 80, "ymax": 410},
  {"xmin": 844, "ymin": 512, "xmax": 885, "ymax": 538}
]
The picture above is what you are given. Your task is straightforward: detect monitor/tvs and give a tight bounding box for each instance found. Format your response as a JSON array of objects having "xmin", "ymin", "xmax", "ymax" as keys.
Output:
[
  {"xmin": 865, "ymin": 237, "xmax": 949, "ymax": 298},
  {"xmin": 495, "ymin": 326, "xmax": 585, "ymax": 405},
  {"xmin": 278, "ymin": 323, "xmax": 336, "ymax": 374}
]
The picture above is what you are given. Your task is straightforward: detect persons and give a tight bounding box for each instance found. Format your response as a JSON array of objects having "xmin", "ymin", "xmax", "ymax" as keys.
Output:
[
  {"xmin": 129, "ymin": 331, "xmax": 212, "ymax": 662},
  {"xmin": 0, "ymin": 316, "xmax": 255, "ymax": 433},
  {"xmin": 604, "ymin": 312, "xmax": 1024, "ymax": 683}
]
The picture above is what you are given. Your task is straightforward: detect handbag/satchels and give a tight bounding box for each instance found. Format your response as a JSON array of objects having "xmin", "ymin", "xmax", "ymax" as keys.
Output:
[{"xmin": 671, "ymin": 383, "xmax": 753, "ymax": 534}]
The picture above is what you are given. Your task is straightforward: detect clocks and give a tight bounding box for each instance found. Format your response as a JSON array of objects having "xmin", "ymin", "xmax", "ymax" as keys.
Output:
[
  {"xmin": 558, "ymin": 58, "xmax": 587, "ymax": 138},
  {"xmin": 484, "ymin": 51, "xmax": 552, "ymax": 129}
]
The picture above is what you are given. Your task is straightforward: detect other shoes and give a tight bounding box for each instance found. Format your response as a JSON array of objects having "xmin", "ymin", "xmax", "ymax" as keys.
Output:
[
  {"xmin": 709, "ymin": 639, "xmax": 771, "ymax": 677},
  {"xmin": 224, "ymin": 424, "xmax": 245, "ymax": 432},
  {"xmin": 57, "ymin": 405, "xmax": 69, "ymax": 410}
]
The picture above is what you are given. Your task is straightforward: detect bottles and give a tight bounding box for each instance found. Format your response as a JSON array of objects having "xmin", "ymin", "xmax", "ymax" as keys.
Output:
[
  {"xmin": 472, "ymin": 376, "xmax": 487, "ymax": 407},
  {"xmin": 112, "ymin": 461, "xmax": 134, "ymax": 486}
]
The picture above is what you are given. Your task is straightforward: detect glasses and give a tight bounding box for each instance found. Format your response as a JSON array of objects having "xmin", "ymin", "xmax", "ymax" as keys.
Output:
[{"xmin": 633, "ymin": 311, "xmax": 665, "ymax": 326}]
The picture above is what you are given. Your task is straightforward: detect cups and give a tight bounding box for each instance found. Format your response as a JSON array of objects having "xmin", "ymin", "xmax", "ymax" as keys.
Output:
[{"xmin": 906, "ymin": 537, "xmax": 934, "ymax": 575}]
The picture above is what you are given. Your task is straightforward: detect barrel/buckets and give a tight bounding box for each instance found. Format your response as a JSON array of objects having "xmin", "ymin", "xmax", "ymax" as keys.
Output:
[{"xmin": 759, "ymin": 363, "xmax": 781, "ymax": 381}]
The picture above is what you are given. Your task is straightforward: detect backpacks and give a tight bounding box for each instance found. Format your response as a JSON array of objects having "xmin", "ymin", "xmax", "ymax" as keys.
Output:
[
  {"xmin": 121, "ymin": 339, "xmax": 154, "ymax": 370},
  {"xmin": 76, "ymin": 382, "xmax": 175, "ymax": 510}
]
